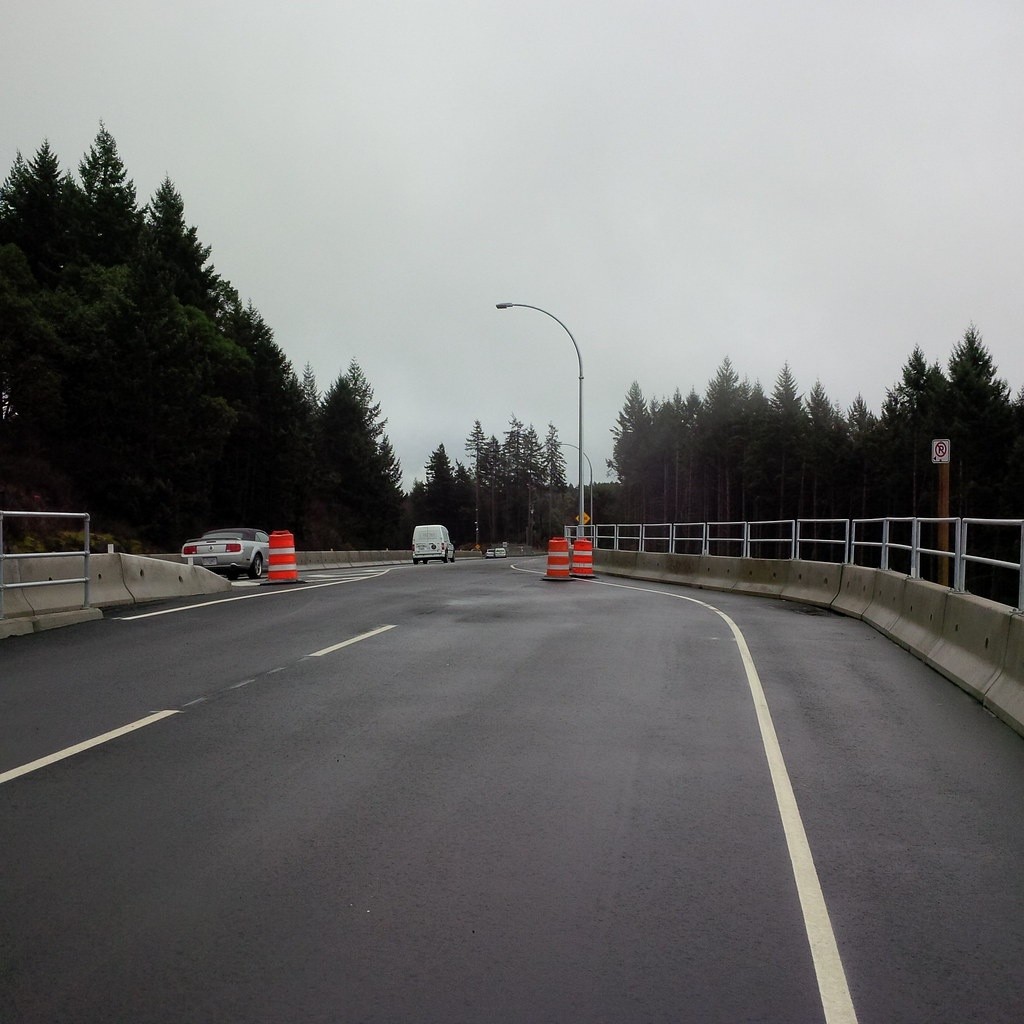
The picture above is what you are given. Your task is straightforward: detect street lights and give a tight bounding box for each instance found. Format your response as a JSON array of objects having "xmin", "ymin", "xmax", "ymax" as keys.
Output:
[
  {"xmin": 553, "ymin": 442, "xmax": 593, "ymax": 544},
  {"xmin": 496, "ymin": 303, "xmax": 588, "ymax": 540}
]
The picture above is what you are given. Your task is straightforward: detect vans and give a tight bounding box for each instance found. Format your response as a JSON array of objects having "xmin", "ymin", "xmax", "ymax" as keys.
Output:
[{"xmin": 413, "ymin": 524, "xmax": 456, "ymax": 565}]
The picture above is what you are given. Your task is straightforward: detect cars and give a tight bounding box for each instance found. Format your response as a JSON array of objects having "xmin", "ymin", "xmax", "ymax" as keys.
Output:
[
  {"xmin": 180, "ymin": 527, "xmax": 270, "ymax": 580},
  {"xmin": 485, "ymin": 549, "xmax": 495, "ymax": 559},
  {"xmin": 494, "ymin": 547, "xmax": 505, "ymax": 560}
]
